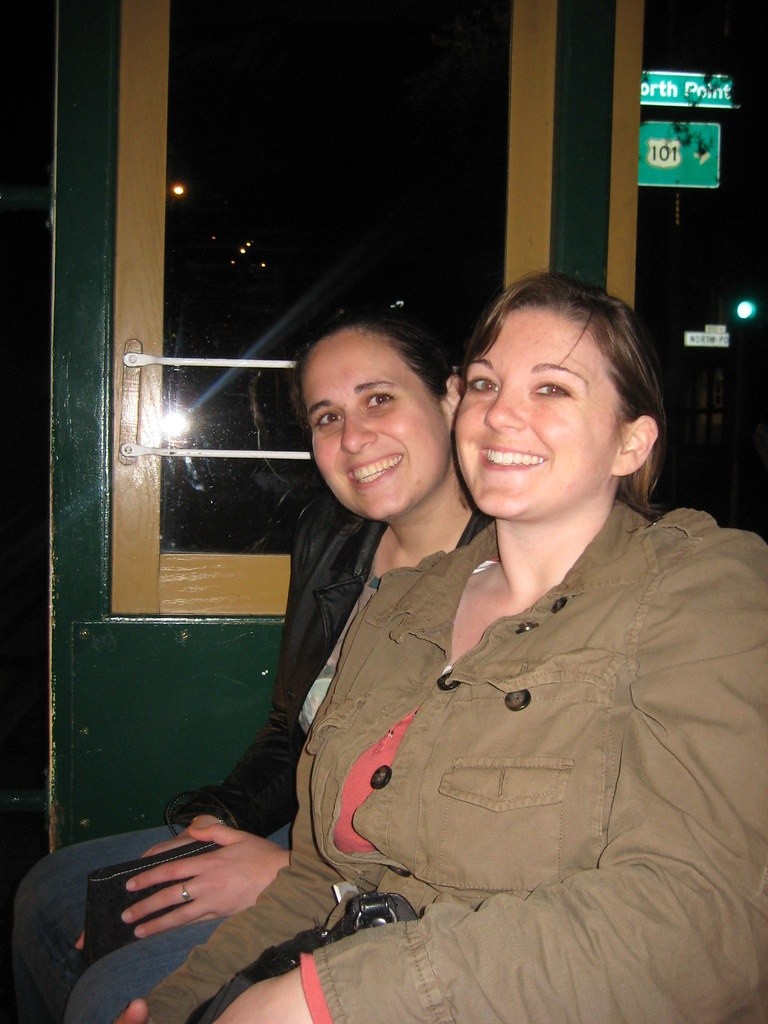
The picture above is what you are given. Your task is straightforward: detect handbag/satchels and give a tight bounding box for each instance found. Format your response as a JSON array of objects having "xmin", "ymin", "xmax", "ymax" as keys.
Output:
[
  {"xmin": 185, "ymin": 891, "xmax": 421, "ymax": 1024},
  {"xmin": 87, "ymin": 790, "xmax": 245, "ymax": 968}
]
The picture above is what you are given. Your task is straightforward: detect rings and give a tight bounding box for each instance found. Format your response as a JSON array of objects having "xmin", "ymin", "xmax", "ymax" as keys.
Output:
[{"xmin": 181, "ymin": 881, "xmax": 192, "ymax": 902}]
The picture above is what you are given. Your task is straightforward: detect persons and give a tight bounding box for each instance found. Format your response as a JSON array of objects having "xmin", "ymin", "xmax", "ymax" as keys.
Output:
[
  {"xmin": 113, "ymin": 272, "xmax": 768, "ymax": 1024},
  {"xmin": 247, "ymin": 357, "xmax": 326, "ymax": 553},
  {"xmin": 10, "ymin": 320, "xmax": 492, "ymax": 1024}
]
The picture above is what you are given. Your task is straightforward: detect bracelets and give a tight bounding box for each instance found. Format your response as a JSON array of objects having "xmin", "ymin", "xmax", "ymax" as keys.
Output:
[{"xmin": 190, "ymin": 805, "xmax": 234, "ymax": 827}]
